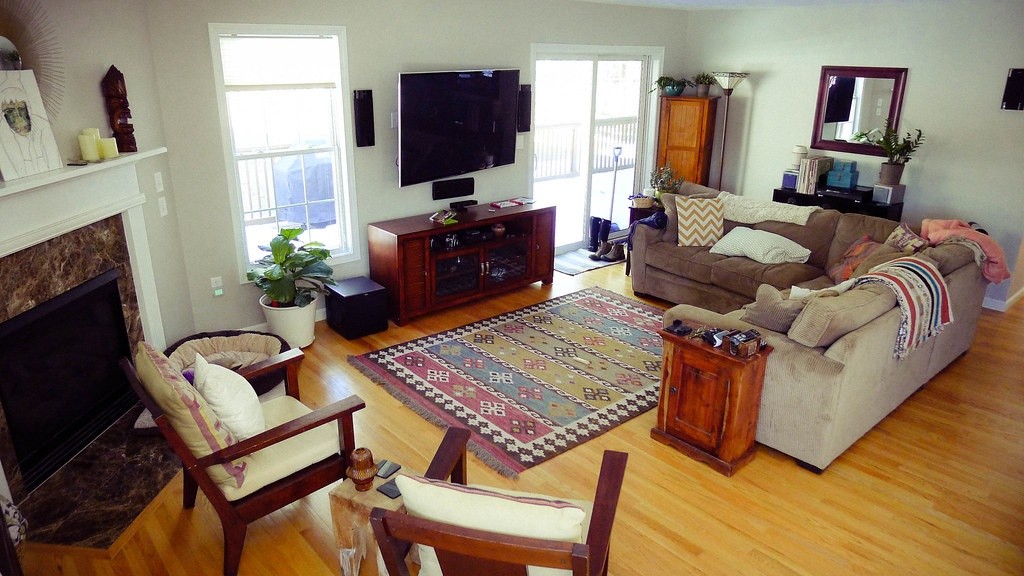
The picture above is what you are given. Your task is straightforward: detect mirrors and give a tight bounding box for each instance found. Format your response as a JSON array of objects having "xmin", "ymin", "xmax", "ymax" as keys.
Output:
[{"xmin": 810, "ymin": 65, "xmax": 909, "ymax": 158}]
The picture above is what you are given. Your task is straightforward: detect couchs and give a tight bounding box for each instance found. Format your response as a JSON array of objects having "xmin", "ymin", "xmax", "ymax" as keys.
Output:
[{"xmin": 629, "ymin": 180, "xmax": 990, "ymax": 477}]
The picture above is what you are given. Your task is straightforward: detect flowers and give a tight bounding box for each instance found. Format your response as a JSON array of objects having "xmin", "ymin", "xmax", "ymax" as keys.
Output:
[{"xmin": 651, "ymin": 161, "xmax": 684, "ymax": 190}]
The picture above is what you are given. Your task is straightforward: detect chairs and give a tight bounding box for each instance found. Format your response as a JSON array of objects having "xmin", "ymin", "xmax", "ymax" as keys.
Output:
[
  {"xmin": 119, "ymin": 338, "xmax": 367, "ymax": 576},
  {"xmin": 372, "ymin": 426, "xmax": 629, "ymax": 576}
]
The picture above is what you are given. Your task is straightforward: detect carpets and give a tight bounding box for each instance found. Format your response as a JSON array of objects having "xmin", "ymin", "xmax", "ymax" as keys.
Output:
[
  {"xmin": 554, "ymin": 247, "xmax": 628, "ymax": 276},
  {"xmin": 346, "ymin": 282, "xmax": 673, "ymax": 480}
]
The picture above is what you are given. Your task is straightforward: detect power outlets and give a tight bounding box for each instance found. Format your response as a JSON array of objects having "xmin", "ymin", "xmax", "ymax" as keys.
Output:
[
  {"xmin": 210, "ymin": 276, "xmax": 223, "ymax": 289},
  {"xmin": 391, "ymin": 112, "xmax": 398, "ymax": 129},
  {"xmin": 154, "ymin": 172, "xmax": 164, "ymax": 193},
  {"xmin": 158, "ymin": 196, "xmax": 168, "ymax": 218},
  {"xmin": 877, "ymin": 99, "xmax": 882, "ymax": 107},
  {"xmin": 876, "ymin": 108, "xmax": 881, "ymax": 116}
]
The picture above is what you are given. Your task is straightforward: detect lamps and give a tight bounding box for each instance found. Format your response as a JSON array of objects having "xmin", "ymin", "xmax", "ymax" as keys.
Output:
[
  {"xmin": 711, "ymin": 71, "xmax": 750, "ymax": 192},
  {"xmin": 347, "ymin": 447, "xmax": 380, "ymax": 492}
]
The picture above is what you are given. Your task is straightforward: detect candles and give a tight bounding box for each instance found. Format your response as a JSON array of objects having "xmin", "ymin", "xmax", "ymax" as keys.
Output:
[{"xmin": 77, "ymin": 128, "xmax": 119, "ymax": 162}]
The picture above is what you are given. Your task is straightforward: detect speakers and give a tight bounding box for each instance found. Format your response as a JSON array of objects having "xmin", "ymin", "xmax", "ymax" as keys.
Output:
[
  {"xmin": 517, "ymin": 84, "xmax": 532, "ymax": 132},
  {"xmin": 432, "ymin": 177, "xmax": 475, "ymax": 200},
  {"xmin": 354, "ymin": 90, "xmax": 376, "ymax": 147},
  {"xmin": 324, "ymin": 276, "xmax": 389, "ymax": 341}
]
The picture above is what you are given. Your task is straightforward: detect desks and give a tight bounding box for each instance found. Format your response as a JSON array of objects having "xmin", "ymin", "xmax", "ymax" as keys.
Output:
[{"xmin": 329, "ymin": 464, "xmax": 423, "ymax": 576}]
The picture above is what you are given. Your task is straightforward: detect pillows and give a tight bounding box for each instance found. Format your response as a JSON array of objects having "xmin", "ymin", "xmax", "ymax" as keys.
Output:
[
  {"xmin": 709, "ymin": 226, "xmax": 811, "ymax": 264},
  {"xmin": 882, "ymin": 221, "xmax": 929, "ymax": 256},
  {"xmin": 191, "ymin": 352, "xmax": 265, "ymax": 458},
  {"xmin": 788, "ymin": 277, "xmax": 858, "ymax": 299},
  {"xmin": 675, "ymin": 195, "xmax": 724, "ymax": 246},
  {"xmin": 659, "ymin": 193, "xmax": 717, "ymax": 243},
  {"xmin": 848, "ymin": 243, "xmax": 914, "ymax": 278},
  {"xmin": 739, "ymin": 283, "xmax": 839, "ymax": 335},
  {"xmin": 825, "ymin": 231, "xmax": 883, "ymax": 285}
]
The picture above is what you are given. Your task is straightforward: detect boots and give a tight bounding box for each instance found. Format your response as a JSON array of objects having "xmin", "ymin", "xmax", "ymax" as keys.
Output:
[
  {"xmin": 588, "ymin": 216, "xmax": 601, "ymax": 251},
  {"xmin": 598, "ymin": 220, "xmax": 611, "ymax": 243},
  {"xmin": 588, "ymin": 240, "xmax": 613, "ymax": 261},
  {"xmin": 600, "ymin": 244, "xmax": 625, "ymax": 262}
]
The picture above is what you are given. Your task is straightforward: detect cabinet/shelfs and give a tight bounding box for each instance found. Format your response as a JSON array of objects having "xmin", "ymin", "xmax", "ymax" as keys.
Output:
[
  {"xmin": 660, "ymin": 94, "xmax": 722, "ymax": 188},
  {"xmin": 772, "ymin": 186, "xmax": 904, "ymax": 222},
  {"xmin": 628, "ymin": 206, "xmax": 667, "ymax": 228},
  {"xmin": 324, "ymin": 275, "xmax": 389, "ymax": 341},
  {"xmin": 367, "ymin": 196, "xmax": 557, "ymax": 328},
  {"xmin": 651, "ymin": 318, "xmax": 776, "ymax": 477}
]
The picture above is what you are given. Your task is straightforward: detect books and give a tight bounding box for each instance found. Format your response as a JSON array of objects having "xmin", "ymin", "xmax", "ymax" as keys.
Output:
[
  {"xmin": 797, "ymin": 156, "xmax": 834, "ymax": 195},
  {"xmin": 429, "ymin": 210, "xmax": 458, "ymax": 225}
]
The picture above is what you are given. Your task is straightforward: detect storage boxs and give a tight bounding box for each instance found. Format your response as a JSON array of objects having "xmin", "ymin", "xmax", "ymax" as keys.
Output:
[
  {"xmin": 825, "ymin": 161, "xmax": 859, "ymax": 188},
  {"xmin": 722, "ymin": 330, "xmax": 761, "ymax": 359}
]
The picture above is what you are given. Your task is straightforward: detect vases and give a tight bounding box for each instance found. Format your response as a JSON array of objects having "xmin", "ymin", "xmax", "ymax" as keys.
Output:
[{"xmin": 655, "ymin": 188, "xmax": 674, "ymax": 201}]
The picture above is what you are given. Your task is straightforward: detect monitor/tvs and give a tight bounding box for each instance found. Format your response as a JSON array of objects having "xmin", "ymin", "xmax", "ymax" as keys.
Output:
[{"xmin": 398, "ymin": 68, "xmax": 519, "ymax": 188}]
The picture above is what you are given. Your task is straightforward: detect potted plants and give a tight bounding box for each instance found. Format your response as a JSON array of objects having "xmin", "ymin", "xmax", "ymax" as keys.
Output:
[
  {"xmin": 854, "ymin": 119, "xmax": 926, "ymax": 186},
  {"xmin": 691, "ymin": 71, "xmax": 717, "ymax": 98},
  {"xmin": 648, "ymin": 76, "xmax": 698, "ymax": 96},
  {"xmin": 248, "ymin": 228, "xmax": 338, "ymax": 352}
]
